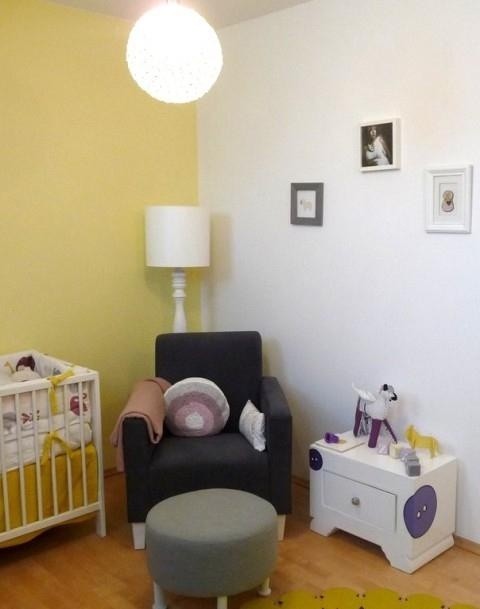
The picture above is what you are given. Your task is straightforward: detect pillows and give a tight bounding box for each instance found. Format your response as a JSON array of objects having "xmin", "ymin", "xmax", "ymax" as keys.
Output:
[
  {"xmin": 238, "ymin": 400, "xmax": 266, "ymax": 453},
  {"xmin": 162, "ymin": 377, "xmax": 230, "ymax": 439}
]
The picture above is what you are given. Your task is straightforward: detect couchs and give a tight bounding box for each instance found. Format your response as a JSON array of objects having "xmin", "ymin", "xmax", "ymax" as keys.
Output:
[{"xmin": 123, "ymin": 331, "xmax": 292, "ymax": 552}]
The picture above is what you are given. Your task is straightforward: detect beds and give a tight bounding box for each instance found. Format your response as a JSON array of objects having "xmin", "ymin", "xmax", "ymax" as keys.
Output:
[{"xmin": 0, "ymin": 348, "xmax": 107, "ymax": 550}]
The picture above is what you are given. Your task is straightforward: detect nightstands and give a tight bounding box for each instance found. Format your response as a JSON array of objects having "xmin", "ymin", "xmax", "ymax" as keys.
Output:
[{"xmin": 310, "ymin": 429, "xmax": 458, "ymax": 573}]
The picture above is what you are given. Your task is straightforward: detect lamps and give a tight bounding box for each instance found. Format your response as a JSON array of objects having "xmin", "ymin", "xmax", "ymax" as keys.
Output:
[
  {"xmin": 142, "ymin": 205, "xmax": 212, "ymax": 334},
  {"xmin": 126, "ymin": 5, "xmax": 225, "ymax": 107}
]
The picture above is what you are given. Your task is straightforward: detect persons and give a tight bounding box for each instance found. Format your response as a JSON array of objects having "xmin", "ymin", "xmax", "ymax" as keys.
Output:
[
  {"xmin": 11, "ymin": 356, "xmax": 40, "ymax": 383},
  {"xmin": 363, "ymin": 127, "xmax": 390, "ymax": 165}
]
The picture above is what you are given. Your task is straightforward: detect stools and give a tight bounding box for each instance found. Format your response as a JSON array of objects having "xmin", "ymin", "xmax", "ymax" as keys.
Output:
[{"xmin": 138, "ymin": 488, "xmax": 278, "ymax": 609}]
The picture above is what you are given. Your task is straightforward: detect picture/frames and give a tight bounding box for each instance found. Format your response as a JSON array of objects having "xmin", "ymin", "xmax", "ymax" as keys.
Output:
[
  {"xmin": 423, "ymin": 164, "xmax": 472, "ymax": 236},
  {"xmin": 289, "ymin": 182, "xmax": 323, "ymax": 226},
  {"xmin": 358, "ymin": 118, "xmax": 401, "ymax": 173}
]
